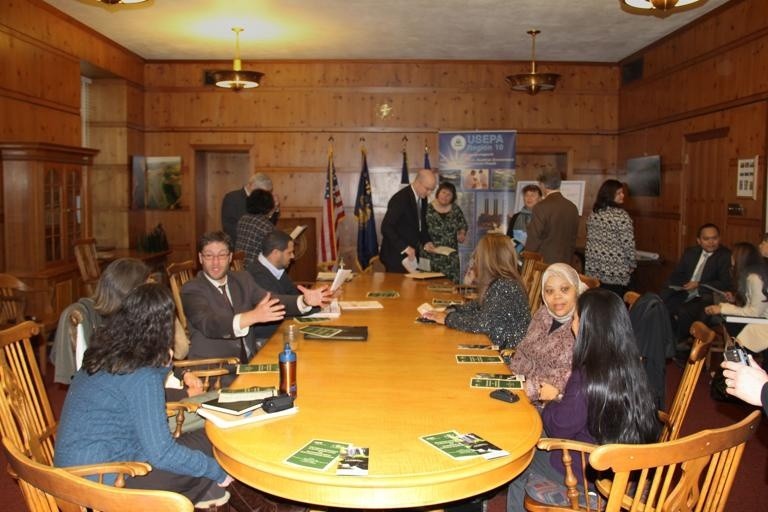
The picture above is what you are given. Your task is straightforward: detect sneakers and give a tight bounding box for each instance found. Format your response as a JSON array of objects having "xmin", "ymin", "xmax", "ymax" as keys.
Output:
[{"xmin": 524, "ymin": 480, "xmax": 605, "ymax": 510}]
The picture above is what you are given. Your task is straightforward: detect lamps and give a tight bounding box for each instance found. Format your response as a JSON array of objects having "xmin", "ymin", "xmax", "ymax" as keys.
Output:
[
  {"xmin": 505, "ymin": 30, "xmax": 560, "ymax": 95},
  {"xmin": 620, "ymin": 0, "xmax": 708, "ymax": 18},
  {"xmin": 213, "ymin": 27, "xmax": 264, "ymax": 91},
  {"xmin": 76, "ymin": 0, "xmax": 154, "ymax": 11}
]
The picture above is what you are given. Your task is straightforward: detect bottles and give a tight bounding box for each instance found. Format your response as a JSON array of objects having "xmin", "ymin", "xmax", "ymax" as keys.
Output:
[{"xmin": 279, "ymin": 342, "xmax": 297, "ymax": 400}]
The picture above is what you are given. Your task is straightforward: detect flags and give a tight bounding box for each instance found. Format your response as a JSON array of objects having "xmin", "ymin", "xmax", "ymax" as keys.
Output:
[
  {"xmin": 400, "ymin": 152, "xmax": 409, "ymax": 188},
  {"xmin": 424, "ymin": 154, "xmax": 431, "ymax": 169},
  {"xmin": 353, "ymin": 147, "xmax": 379, "ymax": 274},
  {"xmin": 317, "ymin": 144, "xmax": 346, "ymax": 273}
]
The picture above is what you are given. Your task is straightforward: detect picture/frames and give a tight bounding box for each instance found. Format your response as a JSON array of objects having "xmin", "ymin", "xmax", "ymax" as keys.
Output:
[{"xmin": 736, "ymin": 155, "xmax": 759, "ymax": 201}]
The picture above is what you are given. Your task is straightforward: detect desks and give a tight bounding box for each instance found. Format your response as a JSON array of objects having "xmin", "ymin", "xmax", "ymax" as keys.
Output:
[
  {"xmin": 206, "ymin": 272, "xmax": 541, "ymax": 512},
  {"xmin": 98, "ymin": 248, "xmax": 171, "ymax": 273}
]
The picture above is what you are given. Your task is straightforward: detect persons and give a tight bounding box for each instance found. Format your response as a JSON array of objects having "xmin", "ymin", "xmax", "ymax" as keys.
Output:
[
  {"xmin": 234, "ymin": 190, "xmax": 276, "ymax": 271},
  {"xmin": 379, "ymin": 168, "xmax": 436, "ymax": 272},
  {"xmin": 662, "ymin": 222, "xmax": 767, "ymax": 419},
  {"xmin": 422, "ymin": 234, "xmax": 532, "ymax": 350},
  {"xmin": 506, "ymin": 286, "xmax": 657, "ymax": 512},
  {"xmin": 52, "ymin": 284, "xmax": 235, "ymax": 510},
  {"xmin": 426, "ymin": 182, "xmax": 468, "ymax": 283},
  {"xmin": 94, "ymin": 256, "xmax": 219, "ymax": 435},
  {"xmin": 181, "ymin": 229, "xmax": 333, "ymax": 385},
  {"xmin": 221, "ymin": 171, "xmax": 280, "ymax": 248},
  {"xmin": 501, "ymin": 264, "xmax": 588, "ymax": 401},
  {"xmin": 248, "ymin": 231, "xmax": 343, "ymax": 351},
  {"xmin": 468, "ymin": 169, "xmax": 489, "ymax": 190},
  {"xmin": 507, "ymin": 172, "xmax": 639, "ymax": 294}
]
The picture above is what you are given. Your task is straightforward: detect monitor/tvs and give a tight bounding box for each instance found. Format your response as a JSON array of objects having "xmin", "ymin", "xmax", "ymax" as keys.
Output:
[{"xmin": 624, "ymin": 155, "xmax": 661, "ymax": 197}]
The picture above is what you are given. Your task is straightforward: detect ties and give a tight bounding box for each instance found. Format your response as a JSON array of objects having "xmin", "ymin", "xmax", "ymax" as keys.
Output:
[
  {"xmin": 695, "ymin": 253, "xmax": 708, "ymax": 281},
  {"xmin": 418, "ymin": 196, "xmax": 421, "ymax": 231},
  {"xmin": 219, "ymin": 286, "xmax": 233, "ymax": 312}
]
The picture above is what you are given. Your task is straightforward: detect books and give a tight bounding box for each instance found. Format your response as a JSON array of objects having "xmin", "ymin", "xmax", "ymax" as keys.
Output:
[
  {"xmin": 196, "ymin": 386, "xmax": 298, "ymax": 430},
  {"xmin": 295, "ymin": 299, "xmax": 367, "ymax": 342}
]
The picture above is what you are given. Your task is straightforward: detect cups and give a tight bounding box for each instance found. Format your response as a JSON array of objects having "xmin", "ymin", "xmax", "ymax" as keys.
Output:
[{"xmin": 283, "ymin": 324, "xmax": 298, "ymax": 351}]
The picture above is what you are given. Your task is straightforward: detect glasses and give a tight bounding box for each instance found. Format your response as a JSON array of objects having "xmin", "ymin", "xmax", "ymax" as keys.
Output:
[{"xmin": 201, "ymin": 251, "xmax": 231, "ymax": 260}]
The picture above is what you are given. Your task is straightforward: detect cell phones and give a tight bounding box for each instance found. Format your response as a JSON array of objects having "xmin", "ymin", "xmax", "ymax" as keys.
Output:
[
  {"xmin": 489, "ymin": 389, "xmax": 519, "ymax": 403},
  {"xmin": 722, "ymin": 348, "xmax": 751, "ymax": 367}
]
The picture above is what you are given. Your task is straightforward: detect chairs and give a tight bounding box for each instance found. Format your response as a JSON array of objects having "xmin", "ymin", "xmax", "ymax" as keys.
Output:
[
  {"xmin": 0, "ymin": 273, "xmax": 42, "ymax": 332},
  {"xmin": 168, "ymin": 261, "xmax": 198, "ymax": 331},
  {"xmin": 72, "ymin": 237, "xmax": 162, "ymax": 299},
  {"xmin": 0, "ymin": 322, "xmax": 196, "ymax": 512},
  {"xmin": 231, "ymin": 251, "xmax": 248, "ymax": 272}
]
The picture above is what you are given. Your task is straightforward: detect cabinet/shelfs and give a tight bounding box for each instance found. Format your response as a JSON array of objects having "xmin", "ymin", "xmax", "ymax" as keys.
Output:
[{"xmin": 0, "ymin": 144, "xmax": 101, "ymax": 376}]
